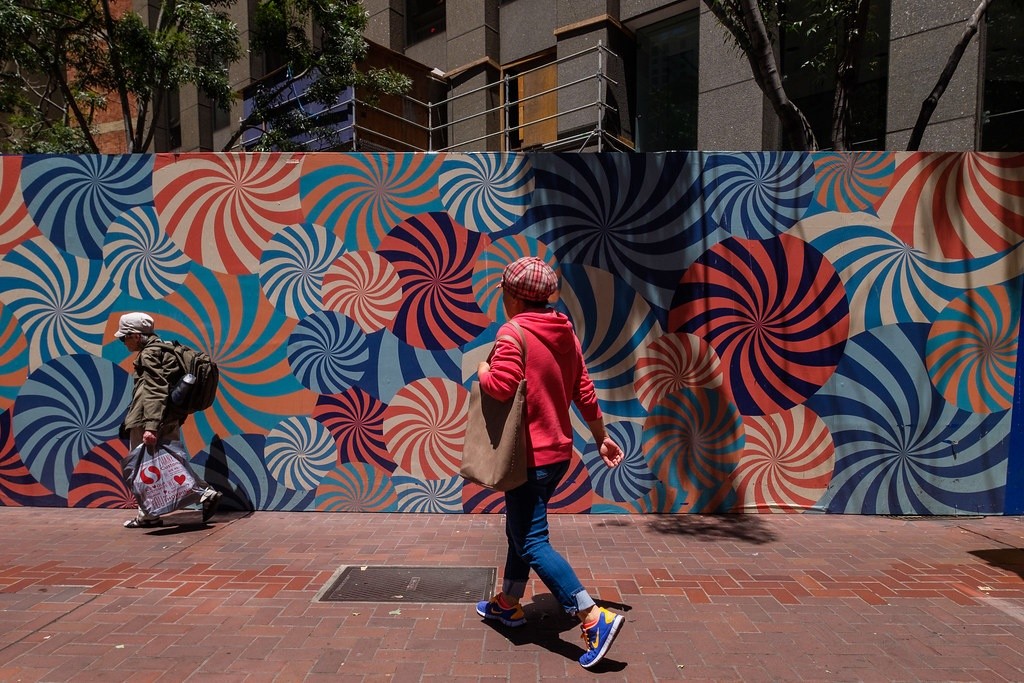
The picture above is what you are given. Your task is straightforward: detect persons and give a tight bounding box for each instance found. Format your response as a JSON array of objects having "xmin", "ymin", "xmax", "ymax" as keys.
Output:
[
  {"xmin": 477, "ymin": 256, "xmax": 626, "ymax": 668},
  {"xmin": 113, "ymin": 312, "xmax": 223, "ymax": 528}
]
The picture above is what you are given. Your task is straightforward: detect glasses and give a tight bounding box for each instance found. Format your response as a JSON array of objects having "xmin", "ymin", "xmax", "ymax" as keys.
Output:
[{"xmin": 119, "ymin": 337, "xmax": 133, "ymax": 342}]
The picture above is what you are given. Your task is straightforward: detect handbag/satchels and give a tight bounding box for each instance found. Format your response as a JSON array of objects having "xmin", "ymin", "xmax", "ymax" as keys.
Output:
[
  {"xmin": 459, "ymin": 378, "xmax": 528, "ymax": 491},
  {"xmin": 122, "ymin": 440, "xmax": 204, "ymax": 520},
  {"xmin": 118, "ymin": 423, "xmax": 131, "ymax": 447}
]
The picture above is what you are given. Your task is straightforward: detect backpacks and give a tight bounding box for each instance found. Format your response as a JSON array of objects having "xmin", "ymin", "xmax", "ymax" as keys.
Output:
[{"xmin": 146, "ymin": 340, "xmax": 219, "ymax": 414}]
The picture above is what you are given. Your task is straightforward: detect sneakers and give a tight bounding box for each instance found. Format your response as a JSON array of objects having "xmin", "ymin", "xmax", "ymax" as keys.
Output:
[
  {"xmin": 579, "ymin": 607, "xmax": 625, "ymax": 669},
  {"xmin": 475, "ymin": 593, "xmax": 527, "ymax": 626}
]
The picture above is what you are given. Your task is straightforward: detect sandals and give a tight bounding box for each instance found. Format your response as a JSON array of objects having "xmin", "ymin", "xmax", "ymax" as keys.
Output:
[
  {"xmin": 125, "ymin": 518, "xmax": 163, "ymax": 528},
  {"xmin": 202, "ymin": 491, "xmax": 223, "ymax": 522}
]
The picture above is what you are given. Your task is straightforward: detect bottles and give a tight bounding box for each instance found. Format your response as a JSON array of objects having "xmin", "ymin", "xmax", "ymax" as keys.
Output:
[{"xmin": 183, "ymin": 374, "xmax": 196, "ymax": 384}]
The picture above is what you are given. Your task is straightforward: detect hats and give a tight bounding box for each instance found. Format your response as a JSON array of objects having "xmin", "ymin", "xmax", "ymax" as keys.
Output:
[
  {"xmin": 113, "ymin": 312, "xmax": 154, "ymax": 338},
  {"xmin": 497, "ymin": 256, "xmax": 558, "ymax": 302}
]
